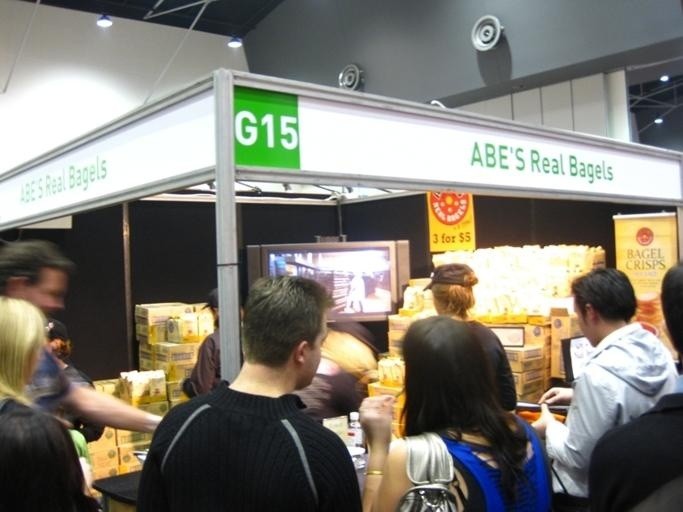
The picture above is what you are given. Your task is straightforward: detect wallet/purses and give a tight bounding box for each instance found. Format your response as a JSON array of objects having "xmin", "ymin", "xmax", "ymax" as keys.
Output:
[{"xmin": 516, "ymin": 401, "xmax": 570, "ymax": 425}]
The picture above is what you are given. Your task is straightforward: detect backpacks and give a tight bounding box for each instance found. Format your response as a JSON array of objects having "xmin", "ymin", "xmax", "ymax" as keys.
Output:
[{"xmin": 397, "ymin": 431, "xmax": 458, "ymax": 512}]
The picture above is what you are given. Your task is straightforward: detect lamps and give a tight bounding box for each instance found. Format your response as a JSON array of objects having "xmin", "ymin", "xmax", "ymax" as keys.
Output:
[
  {"xmin": 227, "ymin": 37, "xmax": 243, "ymax": 49},
  {"xmin": 96, "ymin": 15, "xmax": 113, "ymax": 28}
]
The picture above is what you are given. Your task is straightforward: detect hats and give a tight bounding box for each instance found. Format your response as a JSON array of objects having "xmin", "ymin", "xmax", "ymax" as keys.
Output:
[
  {"xmin": 423, "ymin": 263, "xmax": 478, "ymax": 292},
  {"xmin": 49, "ymin": 317, "xmax": 68, "ymax": 341},
  {"xmin": 201, "ymin": 289, "xmax": 219, "ymax": 310},
  {"xmin": 327, "ymin": 318, "xmax": 380, "ymax": 356}
]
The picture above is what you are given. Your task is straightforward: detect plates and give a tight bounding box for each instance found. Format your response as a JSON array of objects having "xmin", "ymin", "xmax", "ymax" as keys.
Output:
[
  {"xmin": 347, "ymin": 446, "xmax": 365, "ymax": 455},
  {"xmin": 351, "ymin": 457, "xmax": 366, "ymax": 469}
]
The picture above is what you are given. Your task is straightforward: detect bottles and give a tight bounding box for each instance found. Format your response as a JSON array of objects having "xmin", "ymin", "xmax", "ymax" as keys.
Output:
[{"xmin": 346, "ymin": 412, "xmax": 366, "ymax": 470}]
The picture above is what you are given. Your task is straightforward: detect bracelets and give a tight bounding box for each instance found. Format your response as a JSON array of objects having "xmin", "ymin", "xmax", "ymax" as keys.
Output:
[{"xmin": 364, "ymin": 469, "xmax": 383, "ymax": 476}]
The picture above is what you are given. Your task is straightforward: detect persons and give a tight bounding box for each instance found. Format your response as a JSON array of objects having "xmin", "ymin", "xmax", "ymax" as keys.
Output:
[
  {"xmin": 178, "ymin": 288, "xmax": 243, "ymax": 401},
  {"xmin": 45, "ymin": 316, "xmax": 104, "ymax": 440},
  {"xmin": 1, "ymin": 296, "xmax": 104, "ymax": 512},
  {"xmin": 421, "ymin": 263, "xmax": 479, "ymax": 323},
  {"xmin": 359, "ymin": 318, "xmax": 553, "ymax": 512},
  {"xmin": 531, "ymin": 268, "xmax": 677, "ymax": 497},
  {"xmin": 136, "ymin": 276, "xmax": 364, "ymax": 512},
  {"xmin": 346, "ymin": 271, "xmax": 366, "ymax": 313},
  {"xmin": 589, "ymin": 262, "xmax": 682, "ymax": 512},
  {"xmin": 290, "ymin": 354, "xmax": 370, "ymax": 416},
  {"xmin": 1, "ymin": 241, "xmax": 166, "ymax": 433}
]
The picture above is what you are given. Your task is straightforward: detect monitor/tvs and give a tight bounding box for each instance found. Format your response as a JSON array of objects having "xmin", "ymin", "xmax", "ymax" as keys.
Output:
[{"xmin": 246, "ymin": 239, "xmax": 410, "ymax": 324}]
